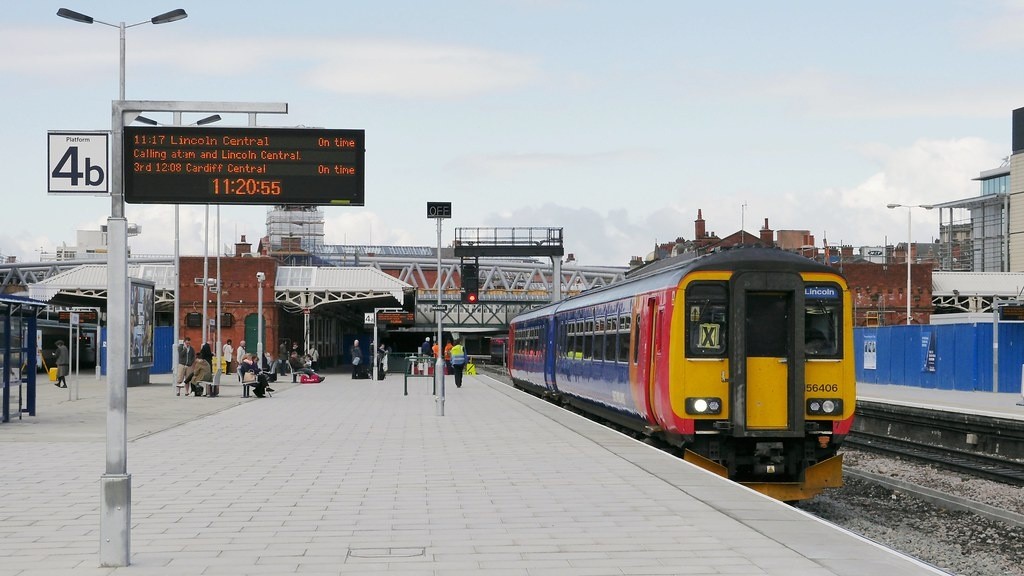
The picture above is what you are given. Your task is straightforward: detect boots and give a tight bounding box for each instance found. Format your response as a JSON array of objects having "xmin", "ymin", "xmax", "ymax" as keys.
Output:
[
  {"xmin": 189, "ymin": 392, "xmax": 195, "ymax": 397},
  {"xmin": 176, "ymin": 383, "xmax": 185, "ymax": 388}
]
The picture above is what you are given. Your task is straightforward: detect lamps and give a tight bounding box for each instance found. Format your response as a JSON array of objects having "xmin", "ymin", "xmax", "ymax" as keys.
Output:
[{"xmin": 953, "ymin": 290, "xmax": 958, "ymax": 294}]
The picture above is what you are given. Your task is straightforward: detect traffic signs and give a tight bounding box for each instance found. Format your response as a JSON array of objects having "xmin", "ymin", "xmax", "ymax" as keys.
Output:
[{"xmin": 431, "ymin": 304, "xmax": 447, "ymax": 312}]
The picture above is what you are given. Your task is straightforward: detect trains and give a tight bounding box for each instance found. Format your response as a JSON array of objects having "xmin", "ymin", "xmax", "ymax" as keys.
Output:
[
  {"xmin": 487, "ymin": 335, "xmax": 511, "ymax": 364},
  {"xmin": 506, "ymin": 242, "xmax": 858, "ymax": 502}
]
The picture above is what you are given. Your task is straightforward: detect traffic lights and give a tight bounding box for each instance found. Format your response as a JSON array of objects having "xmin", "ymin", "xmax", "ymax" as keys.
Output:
[{"xmin": 461, "ymin": 262, "xmax": 479, "ymax": 305}]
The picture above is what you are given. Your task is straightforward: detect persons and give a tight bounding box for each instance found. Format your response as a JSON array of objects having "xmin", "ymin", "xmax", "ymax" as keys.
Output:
[
  {"xmin": 290, "ymin": 342, "xmax": 325, "ymax": 382},
  {"xmin": 237, "ymin": 341, "xmax": 245, "ymax": 382},
  {"xmin": 54, "ymin": 341, "xmax": 68, "ymax": 388},
  {"xmin": 223, "ymin": 339, "xmax": 234, "ymax": 375},
  {"xmin": 200, "ymin": 338, "xmax": 214, "ymax": 374},
  {"xmin": 241, "ymin": 351, "xmax": 277, "ymax": 398},
  {"xmin": 369, "ymin": 338, "xmax": 388, "ymax": 373},
  {"xmin": 450, "ymin": 339, "xmax": 467, "ymax": 388},
  {"xmin": 432, "ymin": 341, "xmax": 438, "ymax": 357},
  {"xmin": 351, "ymin": 340, "xmax": 364, "ymax": 379},
  {"xmin": 177, "ymin": 353, "xmax": 212, "ymax": 397},
  {"xmin": 421, "ymin": 337, "xmax": 432, "ymax": 364},
  {"xmin": 177, "ymin": 337, "xmax": 195, "ymax": 396},
  {"xmin": 444, "ymin": 340, "xmax": 454, "ymax": 375},
  {"xmin": 280, "ymin": 341, "xmax": 289, "ymax": 376}
]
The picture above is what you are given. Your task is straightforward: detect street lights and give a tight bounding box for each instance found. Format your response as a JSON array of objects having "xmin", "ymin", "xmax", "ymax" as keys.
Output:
[
  {"xmin": 132, "ymin": 114, "xmax": 223, "ymax": 389},
  {"xmin": 885, "ymin": 201, "xmax": 935, "ymax": 325},
  {"xmin": 55, "ymin": 6, "xmax": 190, "ymax": 568}
]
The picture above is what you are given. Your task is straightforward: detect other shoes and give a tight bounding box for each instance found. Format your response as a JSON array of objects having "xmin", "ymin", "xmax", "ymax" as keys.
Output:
[
  {"xmin": 177, "ymin": 393, "xmax": 180, "ymax": 396},
  {"xmin": 61, "ymin": 385, "xmax": 67, "ymax": 388},
  {"xmin": 55, "ymin": 383, "xmax": 60, "ymax": 387},
  {"xmin": 253, "ymin": 389, "xmax": 265, "ymax": 397},
  {"xmin": 185, "ymin": 393, "xmax": 189, "ymax": 396},
  {"xmin": 266, "ymin": 388, "xmax": 275, "ymax": 391}
]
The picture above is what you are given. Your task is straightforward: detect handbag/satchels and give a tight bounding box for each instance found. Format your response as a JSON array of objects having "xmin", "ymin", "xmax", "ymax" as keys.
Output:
[
  {"xmin": 301, "ymin": 374, "xmax": 319, "ymax": 383},
  {"xmin": 243, "ymin": 368, "xmax": 256, "ymax": 382}
]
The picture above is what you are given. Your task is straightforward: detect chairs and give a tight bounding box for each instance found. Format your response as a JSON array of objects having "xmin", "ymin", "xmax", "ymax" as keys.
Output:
[
  {"xmin": 285, "ymin": 360, "xmax": 305, "ymax": 383},
  {"xmin": 235, "ymin": 365, "xmax": 259, "ymax": 398},
  {"xmin": 263, "ymin": 359, "xmax": 280, "ymax": 383},
  {"xmin": 197, "ymin": 368, "xmax": 223, "ymax": 397}
]
